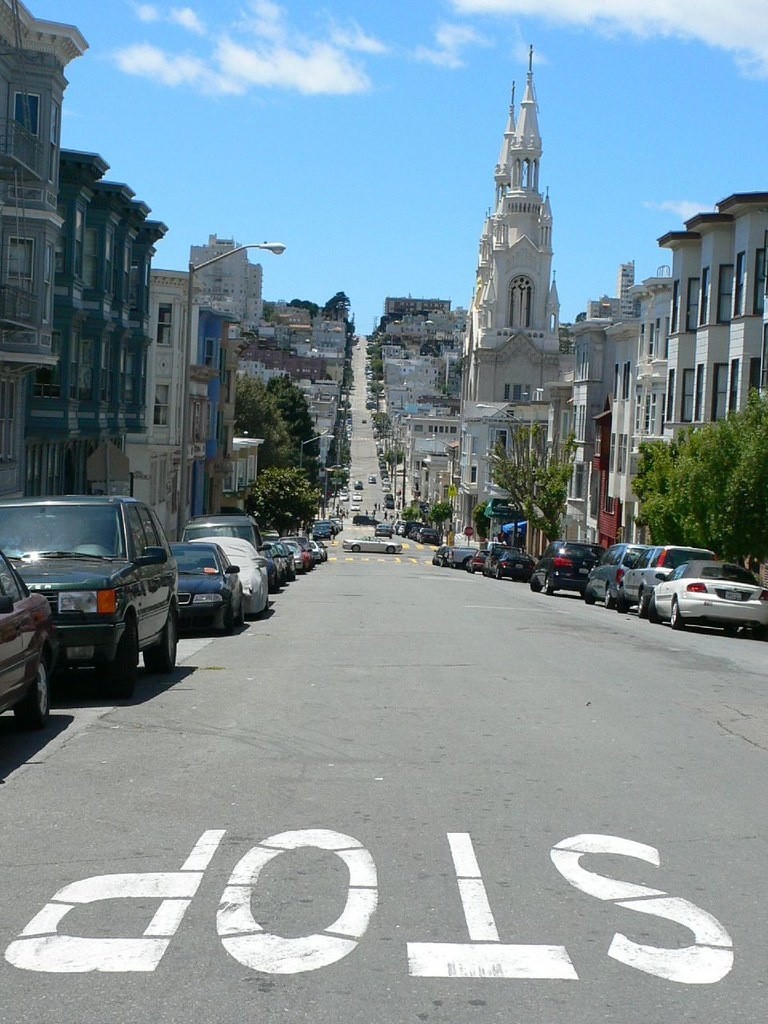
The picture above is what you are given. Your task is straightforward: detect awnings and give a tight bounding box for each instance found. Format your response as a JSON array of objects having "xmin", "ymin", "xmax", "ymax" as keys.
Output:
[{"xmin": 503, "ymin": 520, "xmax": 527, "ymax": 536}]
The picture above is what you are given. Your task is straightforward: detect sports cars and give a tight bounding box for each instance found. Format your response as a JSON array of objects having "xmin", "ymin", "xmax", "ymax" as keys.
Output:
[{"xmin": 342, "ymin": 536, "xmax": 403, "ymax": 554}]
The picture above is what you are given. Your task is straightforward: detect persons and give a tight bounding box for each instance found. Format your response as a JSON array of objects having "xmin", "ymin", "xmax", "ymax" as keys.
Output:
[
  {"xmin": 331, "ymin": 520, "xmax": 337, "ymax": 544},
  {"xmin": 383, "ymin": 510, "xmax": 387, "ymax": 521},
  {"xmin": 366, "ymin": 510, "xmax": 368, "ymax": 515},
  {"xmin": 373, "ymin": 510, "xmax": 375, "ymax": 518},
  {"xmin": 374, "ymin": 502, "xmax": 381, "ymax": 511}
]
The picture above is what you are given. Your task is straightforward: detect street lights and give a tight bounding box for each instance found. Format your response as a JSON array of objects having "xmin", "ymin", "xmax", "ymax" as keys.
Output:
[
  {"xmin": 300, "ymin": 430, "xmax": 336, "ymax": 474},
  {"xmin": 175, "ymin": 239, "xmax": 285, "ymax": 545},
  {"xmin": 475, "ymin": 403, "xmax": 535, "ymax": 555}
]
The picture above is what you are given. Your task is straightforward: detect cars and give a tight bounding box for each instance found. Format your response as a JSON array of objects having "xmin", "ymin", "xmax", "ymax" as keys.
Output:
[
  {"xmin": 1, "ymin": 495, "xmax": 179, "ymax": 698},
  {"xmin": 164, "ymin": 541, "xmax": 243, "ymax": 630},
  {"xmin": 186, "ymin": 535, "xmax": 271, "ymax": 614},
  {"xmin": 530, "ymin": 541, "xmax": 608, "ymax": 599},
  {"xmin": 338, "ymin": 343, "xmax": 396, "ymax": 510},
  {"xmin": 649, "ymin": 558, "xmax": 768, "ymax": 634},
  {"xmin": 432, "ymin": 543, "xmax": 537, "ymax": 581},
  {"xmin": 352, "ymin": 515, "xmax": 440, "ymax": 546},
  {"xmin": 0, "ymin": 547, "xmax": 57, "ymax": 727},
  {"xmin": 616, "ymin": 544, "xmax": 715, "ymax": 618},
  {"xmin": 177, "ymin": 502, "xmax": 344, "ymax": 593},
  {"xmin": 583, "ymin": 543, "xmax": 654, "ymax": 610}
]
